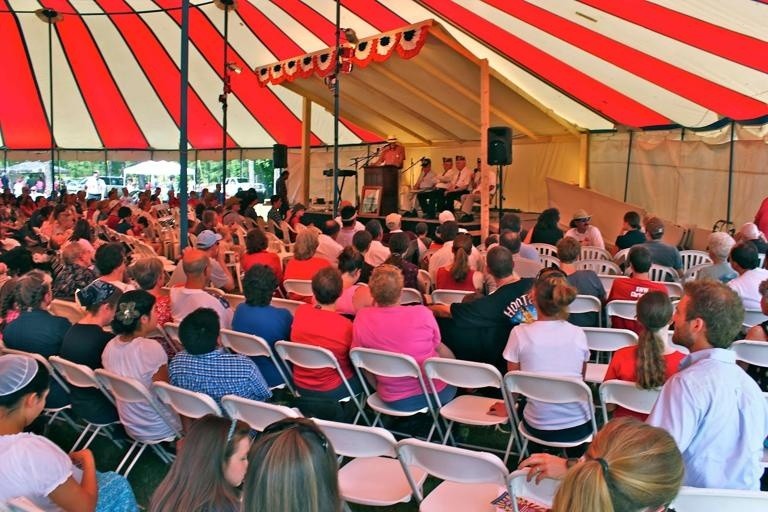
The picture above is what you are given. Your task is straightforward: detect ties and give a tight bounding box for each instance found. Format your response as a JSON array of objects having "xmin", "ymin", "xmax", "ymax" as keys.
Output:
[
  {"xmin": 443, "ymin": 170, "xmax": 447, "ymax": 176},
  {"xmin": 455, "ymin": 172, "xmax": 461, "ymax": 185}
]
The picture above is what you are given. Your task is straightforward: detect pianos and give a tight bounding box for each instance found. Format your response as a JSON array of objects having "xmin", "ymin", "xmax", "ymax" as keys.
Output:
[{"xmin": 323, "ymin": 170, "xmax": 356, "ymax": 176}]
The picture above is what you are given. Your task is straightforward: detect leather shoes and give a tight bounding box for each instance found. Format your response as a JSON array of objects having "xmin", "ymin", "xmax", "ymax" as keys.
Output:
[
  {"xmin": 422, "ymin": 211, "xmax": 436, "ymax": 220},
  {"xmin": 461, "ymin": 214, "xmax": 473, "ymax": 223}
]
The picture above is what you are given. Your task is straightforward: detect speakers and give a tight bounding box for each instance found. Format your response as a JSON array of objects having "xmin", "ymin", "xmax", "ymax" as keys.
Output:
[
  {"xmin": 273, "ymin": 143, "xmax": 288, "ymax": 169},
  {"xmin": 486, "ymin": 126, "xmax": 513, "ymax": 166}
]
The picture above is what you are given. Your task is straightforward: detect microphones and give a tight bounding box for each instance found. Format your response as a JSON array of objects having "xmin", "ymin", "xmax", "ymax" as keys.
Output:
[
  {"xmin": 470, "ymin": 168, "xmax": 478, "ymax": 183},
  {"xmin": 376, "ymin": 148, "xmax": 379, "ymax": 157},
  {"xmin": 383, "ymin": 144, "xmax": 391, "ymax": 148}
]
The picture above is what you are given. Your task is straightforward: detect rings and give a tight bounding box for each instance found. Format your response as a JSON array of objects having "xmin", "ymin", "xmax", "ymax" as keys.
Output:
[{"xmin": 534, "ymin": 467, "xmax": 539, "ymax": 473}]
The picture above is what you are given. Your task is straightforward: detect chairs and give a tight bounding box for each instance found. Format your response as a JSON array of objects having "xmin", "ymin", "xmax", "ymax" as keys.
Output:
[
  {"xmin": 0, "ymin": 204, "xmax": 768, "ymax": 512},
  {"xmin": 472, "ymin": 183, "xmax": 499, "ymax": 222}
]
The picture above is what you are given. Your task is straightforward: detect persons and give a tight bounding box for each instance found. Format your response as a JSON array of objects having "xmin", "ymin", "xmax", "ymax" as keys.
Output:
[
  {"xmin": 2, "ymin": 353, "xmax": 139, "ymax": 511},
  {"xmin": 517, "ymin": 279, "xmax": 767, "ymax": 491},
  {"xmin": 400, "ymin": 159, "xmax": 436, "ymax": 218},
  {"xmin": 551, "ymin": 416, "xmax": 684, "ymax": 509},
  {"xmin": 455, "ymin": 157, "xmax": 496, "ymax": 221},
  {"xmin": 243, "ymin": 418, "xmax": 344, "ymax": 510},
  {"xmin": 439, "ymin": 155, "xmax": 470, "ymax": 219},
  {"xmin": 368, "ymin": 134, "xmax": 405, "ymax": 212},
  {"xmin": 2, "ymin": 170, "xmax": 768, "ymax": 452},
  {"xmin": 418, "ymin": 157, "xmax": 457, "ymax": 219},
  {"xmin": 149, "ymin": 413, "xmax": 253, "ymax": 511}
]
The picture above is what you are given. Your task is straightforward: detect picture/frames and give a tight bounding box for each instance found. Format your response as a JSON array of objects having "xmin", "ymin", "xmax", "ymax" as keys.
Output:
[{"xmin": 357, "ymin": 185, "xmax": 384, "ymax": 217}]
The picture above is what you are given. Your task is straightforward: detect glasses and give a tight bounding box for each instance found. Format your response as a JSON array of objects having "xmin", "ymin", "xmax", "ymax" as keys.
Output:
[
  {"xmin": 576, "ymin": 218, "xmax": 591, "ymax": 223},
  {"xmin": 536, "ymin": 266, "xmax": 567, "ymax": 284}
]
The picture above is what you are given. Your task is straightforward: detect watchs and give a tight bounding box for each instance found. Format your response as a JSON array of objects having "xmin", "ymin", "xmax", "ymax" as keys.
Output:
[{"xmin": 567, "ymin": 458, "xmax": 579, "ymax": 468}]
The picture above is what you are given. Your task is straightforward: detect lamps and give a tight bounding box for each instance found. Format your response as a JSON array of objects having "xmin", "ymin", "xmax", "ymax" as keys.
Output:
[
  {"xmin": 226, "ymin": 63, "xmax": 242, "ymax": 75},
  {"xmin": 344, "ymin": 28, "xmax": 359, "ymax": 46}
]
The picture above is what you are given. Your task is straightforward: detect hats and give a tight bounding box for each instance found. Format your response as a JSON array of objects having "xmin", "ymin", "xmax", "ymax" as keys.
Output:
[
  {"xmin": 442, "ymin": 155, "xmax": 482, "ymax": 165},
  {"xmin": 573, "ymin": 209, "xmax": 593, "ymax": 220},
  {"xmin": 438, "ymin": 210, "xmax": 456, "ymax": 226},
  {"xmin": 196, "ymin": 230, "xmax": 223, "ymax": 250},
  {"xmin": 0, "ymin": 353, "xmax": 38, "ymax": 396},
  {"xmin": 643, "ymin": 217, "xmax": 665, "ymax": 237},
  {"xmin": 385, "ymin": 213, "xmax": 402, "ymax": 230},
  {"xmin": 294, "ymin": 203, "xmax": 307, "ymax": 214},
  {"xmin": 420, "ymin": 159, "xmax": 431, "ymax": 166},
  {"xmin": 384, "ymin": 136, "xmax": 398, "ymax": 144}
]
